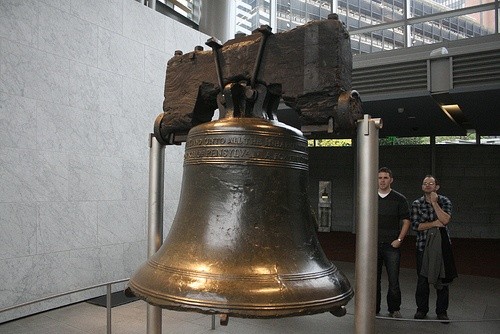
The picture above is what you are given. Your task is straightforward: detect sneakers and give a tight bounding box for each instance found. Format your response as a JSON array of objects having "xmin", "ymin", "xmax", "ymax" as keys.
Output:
[
  {"xmin": 438, "ymin": 314, "xmax": 450, "ymax": 324},
  {"xmin": 390, "ymin": 311, "xmax": 402, "ymax": 318},
  {"xmin": 414, "ymin": 312, "xmax": 427, "ymax": 319}
]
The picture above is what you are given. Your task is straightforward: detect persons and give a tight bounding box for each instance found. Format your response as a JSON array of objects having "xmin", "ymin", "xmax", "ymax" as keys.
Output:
[
  {"xmin": 411, "ymin": 175, "xmax": 453, "ymax": 324},
  {"xmin": 375, "ymin": 166, "xmax": 411, "ymax": 318}
]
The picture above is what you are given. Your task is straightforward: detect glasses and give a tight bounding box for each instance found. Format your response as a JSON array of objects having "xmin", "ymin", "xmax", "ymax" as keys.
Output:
[{"xmin": 423, "ymin": 182, "xmax": 434, "ymax": 185}]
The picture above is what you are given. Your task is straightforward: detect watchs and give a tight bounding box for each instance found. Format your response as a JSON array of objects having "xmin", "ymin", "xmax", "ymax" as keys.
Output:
[{"xmin": 397, "ymin": 238, "xmax": 403, "ymax": 245}]
